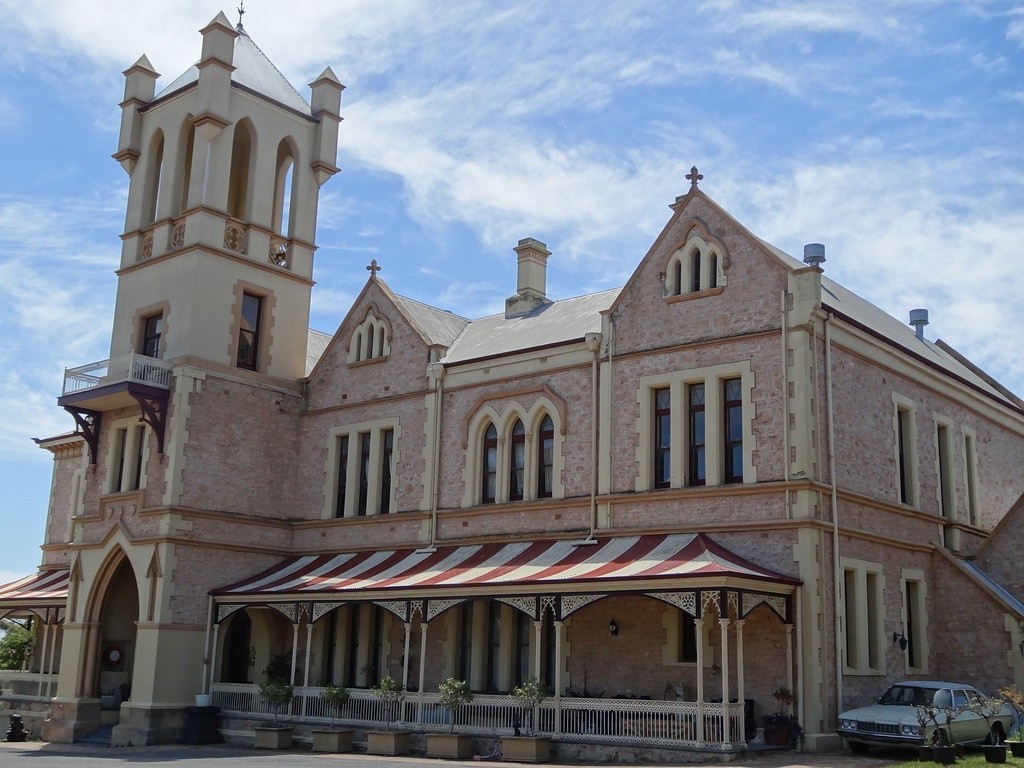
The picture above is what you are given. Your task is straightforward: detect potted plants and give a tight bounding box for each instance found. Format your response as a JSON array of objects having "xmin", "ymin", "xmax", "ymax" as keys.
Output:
[
  {"xmin": 910, "ymin": 704, "xmax": 936, "ymax": 761},
  {"xmin": 499, "ymin": 678, "xmax": 552, "ymax": 762},
  {"xmin": 254, "ymin": 664, "xmax": 296, "ymax": 749},
  {"xmin": 998, "ymin": 684, "xmax": 1024, "ymax": 757},
  {"xmin": 312, "ymin": 683, "xmax": 356, "ymax": 753},
  {"xmin": 368, "ymin": 675, "xmax": 413, "ymax": 754},
  {"xmin": 942, "ymin": 704, "xmax": 963, "ymax": 758},
  {"xmin": 968, "ymin": 693, "xmax": 1007, "ymax": 763},
  {"xmin": 425, "ymin": 679, "xmax": 476, "ymax": 758},
  {"xmin": 97, "ymin": 688, "xmax": 115, "ymax": 708},
  {"xmin": 921, "ymin": 706, "xmax": 956, "ymax": 764},
  {"xmin": 760, "ymin": 686, "xmax": 804, "ymax": 749}
]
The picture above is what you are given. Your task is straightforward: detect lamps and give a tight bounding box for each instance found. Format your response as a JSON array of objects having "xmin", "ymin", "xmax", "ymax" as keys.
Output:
[
  {"xmin": 609, "ymin": 618, "xmax": 619, "ymax": 636},
  {"xmin": 893, "ymin": 632, "xmax": 907, "ymax": 651}
]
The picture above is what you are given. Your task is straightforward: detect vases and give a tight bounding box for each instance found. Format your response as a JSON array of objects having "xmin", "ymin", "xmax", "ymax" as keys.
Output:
[{"xmin": 195, "ymin": 694, "xmax": 212, "ymax": 707}]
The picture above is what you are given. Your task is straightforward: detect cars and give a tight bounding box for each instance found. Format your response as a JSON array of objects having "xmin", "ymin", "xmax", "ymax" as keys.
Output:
[{"xmin": 835, "ymin": 680, "xmax": 1019, "ymax": 761}]
